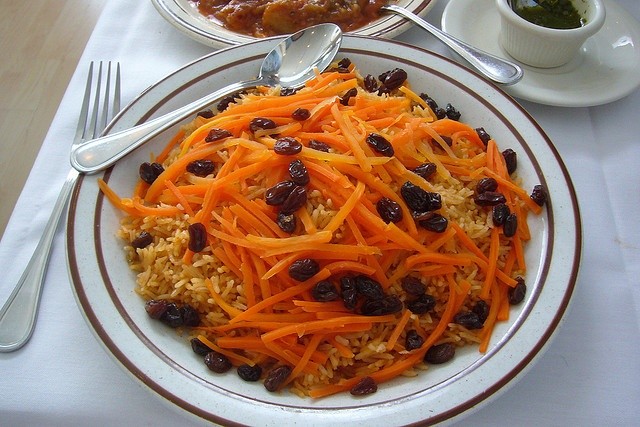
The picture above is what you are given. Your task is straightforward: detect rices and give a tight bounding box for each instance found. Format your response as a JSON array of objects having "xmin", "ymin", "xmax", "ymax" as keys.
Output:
[{"xmin": 114, "ymin": 61, "xmax": 535, "ymax": 399}]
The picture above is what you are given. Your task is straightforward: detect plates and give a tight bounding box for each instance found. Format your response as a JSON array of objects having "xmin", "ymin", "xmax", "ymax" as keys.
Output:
[
  {"xmin": 63, "ymin": 35, "xmax": 583, "ymax": 427},
  {"xmin": 441, "ymin": 1, "xmax": 639, "ymax": 109},
  {"xmin": 150, "ymin": 0, "xmax": 436, "ymax": 50}
]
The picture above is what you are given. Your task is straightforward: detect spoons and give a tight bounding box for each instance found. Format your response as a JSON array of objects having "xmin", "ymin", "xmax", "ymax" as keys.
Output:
[{"xmin": 69, "ymin": 24, "xmax": 344, "ymax": 173}]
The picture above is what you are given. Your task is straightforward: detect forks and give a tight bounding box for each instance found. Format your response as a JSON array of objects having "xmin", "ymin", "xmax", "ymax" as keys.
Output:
[{"xmin": 2, "ymin": 60, "xmax": 123, "ymax": 352}]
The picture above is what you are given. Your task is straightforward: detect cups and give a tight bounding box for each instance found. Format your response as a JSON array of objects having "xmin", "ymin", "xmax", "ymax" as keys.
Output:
[{"xmin": 496, "ymin": 1, "xmax": 607, "ymax": 70}]
